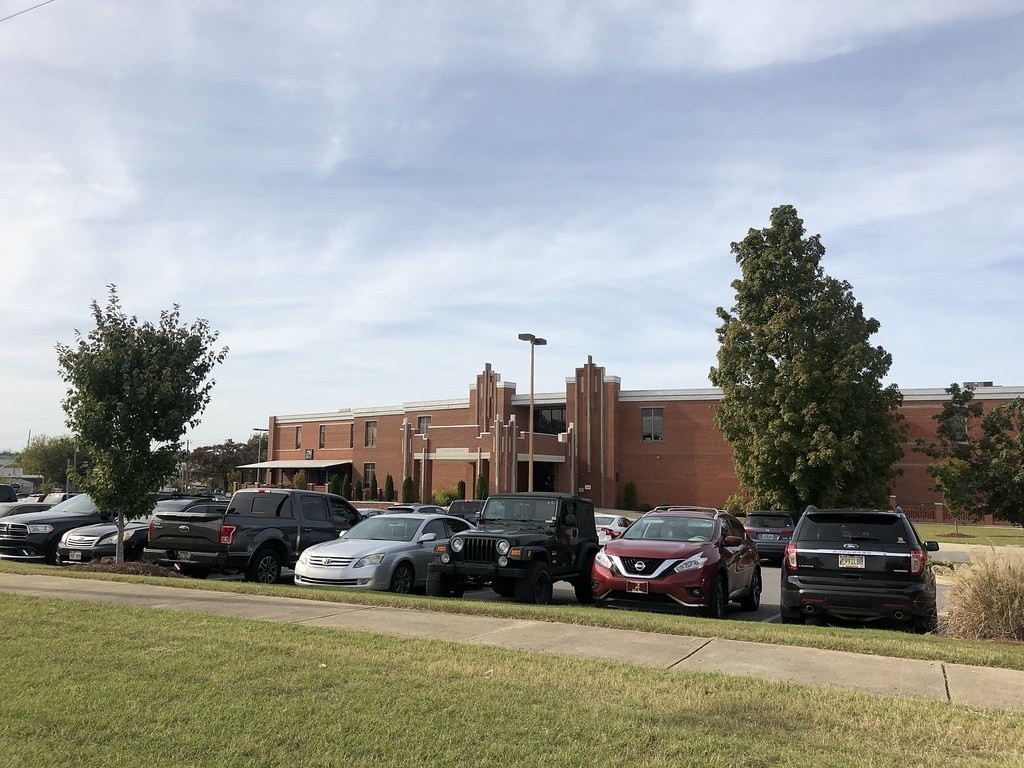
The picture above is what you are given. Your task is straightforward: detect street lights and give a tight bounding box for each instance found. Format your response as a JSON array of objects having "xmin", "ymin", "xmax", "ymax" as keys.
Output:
[
  {"xmin": 252, "ymin": 428, "xmax": 270, "ymax": 482},
  {"xmin": 518, "ymin": 331, "xmax": 547, "ymax": 495}
]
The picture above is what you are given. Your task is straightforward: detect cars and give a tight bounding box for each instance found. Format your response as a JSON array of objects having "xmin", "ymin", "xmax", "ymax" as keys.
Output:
[
  {"xmin": 592, "ymin": 513, "xmax": 635, "ymax": 549},
  {"xmin": 293, "ymin": 512, "xmax": 487, "ymax": 594},
  {"xmin": 54, "ymin": 494, "xmax": 215, "ymax": 568},
  {"xmin": 445, "ymin": 498, "xmax": 487, "ymax": 527},
  {"xmin": 741, "ymin": 510, "xmax": 795, "ymax": 560},
  {"xmin": 0, "ymin": 482, "xmax": 113, "ymax": 567},
  {"xmin": 356, "ymin": 508, "xmax": 385, "ymax": 522},
  {"xmin": 382, "ymin": 502, "xmax": 450, "ymax": 516}
]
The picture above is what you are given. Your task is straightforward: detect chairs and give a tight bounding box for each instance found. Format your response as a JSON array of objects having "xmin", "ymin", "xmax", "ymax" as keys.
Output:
[
  {"xmin": 660, "ymin": 525, "xmax": 677, "ymax": 539},
  {"xmin": 815, "ymin": 524, "xmax": 843, "ymax": 540}
]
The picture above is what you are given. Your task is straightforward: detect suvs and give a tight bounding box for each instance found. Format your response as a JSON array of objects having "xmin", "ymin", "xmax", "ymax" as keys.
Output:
[
  {"xmin": 424, "ymin": 492, "xmax": 599, "ymax": 604},
  {"xmin": 777, "ymin": 504, "xmax": 941, "ymax": 636},
  {"xmin": 589, "ymin": 505, "xmax": 764, "ymax": 621}
]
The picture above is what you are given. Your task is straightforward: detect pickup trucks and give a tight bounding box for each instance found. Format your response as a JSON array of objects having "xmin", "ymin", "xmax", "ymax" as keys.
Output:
[{"xmin": 142, "ymin": 486, "xmax": 363, "ymax": 584}]
